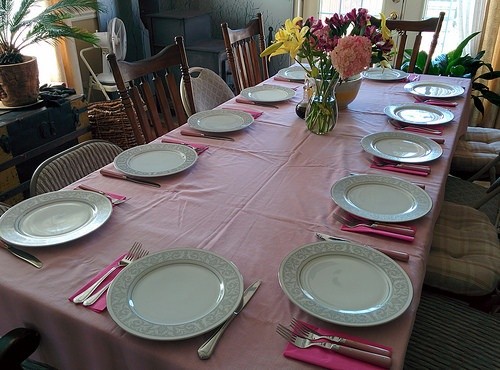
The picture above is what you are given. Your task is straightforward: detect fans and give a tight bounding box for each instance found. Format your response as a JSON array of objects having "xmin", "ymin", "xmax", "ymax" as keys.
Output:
[{"xmin": 91, "ymin": 16, "xmax": 126, "ymax": 86}]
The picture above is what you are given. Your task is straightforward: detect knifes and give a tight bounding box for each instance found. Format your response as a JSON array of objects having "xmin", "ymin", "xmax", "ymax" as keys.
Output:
[
  {"xmin": 274, "ymin": 77, "xmax": 304, "ymax": 84},
  {"xmin": 197, "ymin": 279, "xmax": 263, "ymax": 360},
  {"xmin": 0, "ymin": 239, "xmax": 43, "ymax": 269},
  {"xmin": 236, "ymin": 99, "xmax": 279, "ymax": 109},
  {"xmin": 99, "ymin": 169, "xmax": 163, "ymax": 189},
  {"xmin": 315, "ymin": 232, "xmax": 411, "ymax": 263},
  {"xmin": 181, "ymin": 129, "xmax": 235, "ymax": 142}
]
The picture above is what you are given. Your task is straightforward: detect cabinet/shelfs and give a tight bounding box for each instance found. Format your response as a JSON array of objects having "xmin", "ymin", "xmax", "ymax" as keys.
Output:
[{"xmin": 0, "ymin": 94, "xmax": 93, "ymax": 206}]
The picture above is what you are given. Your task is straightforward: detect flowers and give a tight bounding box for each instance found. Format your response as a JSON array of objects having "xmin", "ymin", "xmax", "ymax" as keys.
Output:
[{"xmin": 259, "ymin": 7, "xmax": 399, "ymax": 134}]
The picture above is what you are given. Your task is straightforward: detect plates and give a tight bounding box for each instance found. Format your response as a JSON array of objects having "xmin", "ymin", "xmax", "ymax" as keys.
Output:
[
  {"xmin": 240, "ymin": 85, "xmax": 296, "ymax": 102},
  {"xmin": 106, "ymin": 247, "xmax": 244, "ymax": 341},
  {"xmin": 0, "ymin": 193, "xmax": 109, "ymax": 247},
  {"xmin": 361, "ymin": 67, "xmax": 408, "ymax": 80},
  {"xmin": 187, "ymin": 109, "xmax": 254, "ymax": 133},
  {"xmin": 114, "ymin": 143, "xmax": 198, "ymax": 178},
  {"xmin": 278, "ymin": 64, "xmax": 315, "ymax": 80},
  {"xmin": 278, "ymin": 81, "xmax": 465, "ymax": 328}
]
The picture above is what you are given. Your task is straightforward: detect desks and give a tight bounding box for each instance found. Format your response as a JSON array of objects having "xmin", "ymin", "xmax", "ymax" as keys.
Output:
[{"xmin": 0, "ymin": 61, "xmax": 474, "ymax": 370}]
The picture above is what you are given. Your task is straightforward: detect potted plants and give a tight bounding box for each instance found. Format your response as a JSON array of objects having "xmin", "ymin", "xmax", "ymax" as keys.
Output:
[{"xmin": 0, "ymin": 0, "xmax": 110, "ymax": 105}]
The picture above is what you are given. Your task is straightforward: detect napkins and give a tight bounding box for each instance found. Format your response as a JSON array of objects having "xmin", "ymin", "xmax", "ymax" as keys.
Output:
[
  {"xmin": 284, "ymin": 319, "xmax": 394, "ymax": 370},
  {"xmin": 68, "ymin": 74, "xmax": 459, "ymax": 313}
]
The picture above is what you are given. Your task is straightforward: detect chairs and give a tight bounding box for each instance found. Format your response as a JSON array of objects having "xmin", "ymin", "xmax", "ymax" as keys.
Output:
[
  {"xmin": 369, "ymin": 11, "xmax": 445, "ymax": 74},
  {"xmin": 403, "ymin": 127, "xmax": 500, "ymax": 370},
  {"xmin": 105, "ymin": 35, "xmax": 196, "ymax": 146},
  {"xmin": 221, "ymin": 12, "xmax": 270, "ymax": 93},
  {"xmin": 29, "ymin": 140, "xmax": 123, "ymax": 197},
  {"xmin": 179, "ymin": 67, "xmax": 236, "ymax": 117},
  {"xmin": 80, "ymin": 47, "xmax": 153, "ymax": 126}
]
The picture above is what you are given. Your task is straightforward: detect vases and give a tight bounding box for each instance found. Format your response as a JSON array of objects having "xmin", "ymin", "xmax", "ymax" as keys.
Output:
[{"xmin": 303, "ymin": 72, "xmax": 340, "ymax": 134}]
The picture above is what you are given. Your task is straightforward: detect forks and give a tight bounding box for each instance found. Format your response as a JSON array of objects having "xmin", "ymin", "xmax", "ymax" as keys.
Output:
[
  {"xmin": 161, "ymin": 139, "xmax": 204, "ymax": 152},
  {"xmin": 366, "ymin": 157, "xmax": 432, "ymax": 174},
  {"xmin": 79, "ymin": 184, "xmax": 132, "ymax": 205},
  {"xmin": 390, "ymin": 119, "xmax": 442, "ymax": 134},
  {"xmin": 275, "ymin": 324, "xmax": 392, "ymax": 369},
  {"xmin": 332, "ymin": 213, "xmax": 416, "ymax": 237},
  {"xmin": 289, "ymin": 318, "xmax": 392, "ymax": 358},
  {"xmin": 82, "ymin": 248, "xmax": 152, "ymax": 306},
  {"xmin": 73, "ymin": 241, "xmax": 143, "ymax": 303},
  {"xmin": 413, "ymin": 95, "xmax": 458, "ymax": 107}
]
